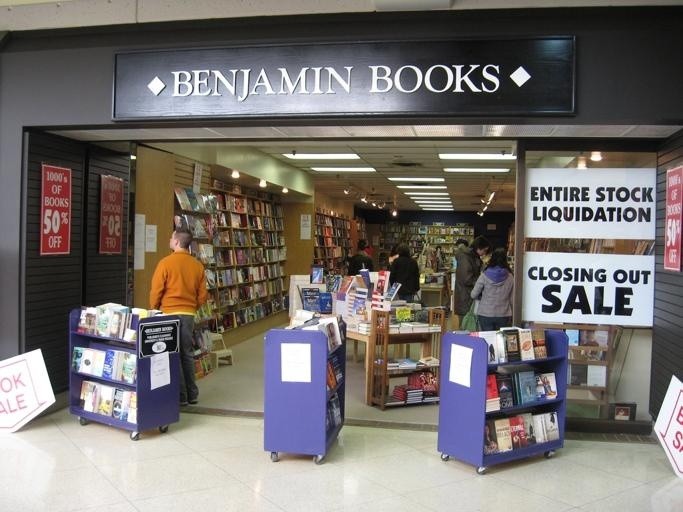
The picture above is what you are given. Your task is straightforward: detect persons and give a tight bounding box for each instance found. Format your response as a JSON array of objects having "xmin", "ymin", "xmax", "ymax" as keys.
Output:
[
  {"xmin": 147, "ymin": 228, "xmax": 207, "ymax": 407},
  {"xmin": 453, "ymin": 236, "xmax": 514, "ymax": 330},
  {"xmin": 346, "ymin": 240, "xmax": 421, "ymax": 303}
]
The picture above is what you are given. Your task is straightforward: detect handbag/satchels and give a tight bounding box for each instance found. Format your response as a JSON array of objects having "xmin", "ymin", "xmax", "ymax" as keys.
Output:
[
  {"xmin": 407, "ymin": 293, "xmax": 424, "ymax": 307},
  {"xmin": 460, "ymin": 299, "xmax": 478, "ymax": 332}
]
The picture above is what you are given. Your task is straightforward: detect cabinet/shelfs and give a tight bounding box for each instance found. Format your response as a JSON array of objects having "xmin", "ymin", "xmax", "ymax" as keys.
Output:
[
  {"xmin": 206, "ymin": 186, "xmax": 353, "ymax": 334},
  {"xmin": 263, "ymin": 320, "xmax": 347, "ymax": 465},
  {"xmin": 68, "ymin": 308, "xmax": 181, "ymax": 440},
  {"xmin": 367, "ymin": 308, "xmax": 446, "ymax": 410},
  {"xmin": 531, "ymin": 321, "xmax": 615, "ymax": 419},
  {"xmin": 437, "ymin": 330, "xmax": 569, "ymax": 475}
]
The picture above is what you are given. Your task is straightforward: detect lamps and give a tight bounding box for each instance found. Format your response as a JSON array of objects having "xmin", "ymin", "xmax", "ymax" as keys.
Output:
[
  {"xmin": 376, "ymin": 225, "xmax": 474, "ymax": 270},
  {"xmin": 343, "ymin": 183, "xmax": 398, "ymax": 217},
  {"xmin": 477, "ymin": 189, "xmax": 496, "ymax": 217}
]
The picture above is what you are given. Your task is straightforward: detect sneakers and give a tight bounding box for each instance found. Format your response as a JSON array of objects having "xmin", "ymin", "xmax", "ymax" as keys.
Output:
[{"xmin": 179, "ymin": 398, "xmax": 198, "ymax": 406}]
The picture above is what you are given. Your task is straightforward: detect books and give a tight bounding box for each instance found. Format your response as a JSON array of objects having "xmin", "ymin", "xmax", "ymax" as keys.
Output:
[
  {"xmin": 565, "ymin": 324, "xmax": 608, "ymax": 386},
  {"xmin": 286, "ymin": 310, "xmax": 348, "ymax": 431},
  {"xmin": 313, "ymin": 214, "xmax": 367, "ymax": 274},
  {"xmin": 506, "ymin": 221, "xmax": 655, "ymax": 260},
  {"xmin": 70, "ymin": 303, "xmax": 168, "ymax": 422},
  {"xmin": 289, "ymin": 269, "xmax": 442, "ymax": 335},
  {"xmin": 373, "ymin": 356, "xmax": 441, "ymax": 407},
  {"xmin": 383, "ymin": 221, "xmax": 475, "ymax": 288},
  {"xmin": 453, "ymin": 327, "xmax": 559, "ymax": 454},
  {"xmin": 175, "ymin": 179, "xmax": 289, "ymax": 334}
]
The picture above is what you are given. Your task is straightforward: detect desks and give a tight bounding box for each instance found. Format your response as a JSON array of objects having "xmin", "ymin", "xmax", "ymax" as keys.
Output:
[{"xmin": 346, "ymin": 329, "xmax": 432, "ymax": 407}]
